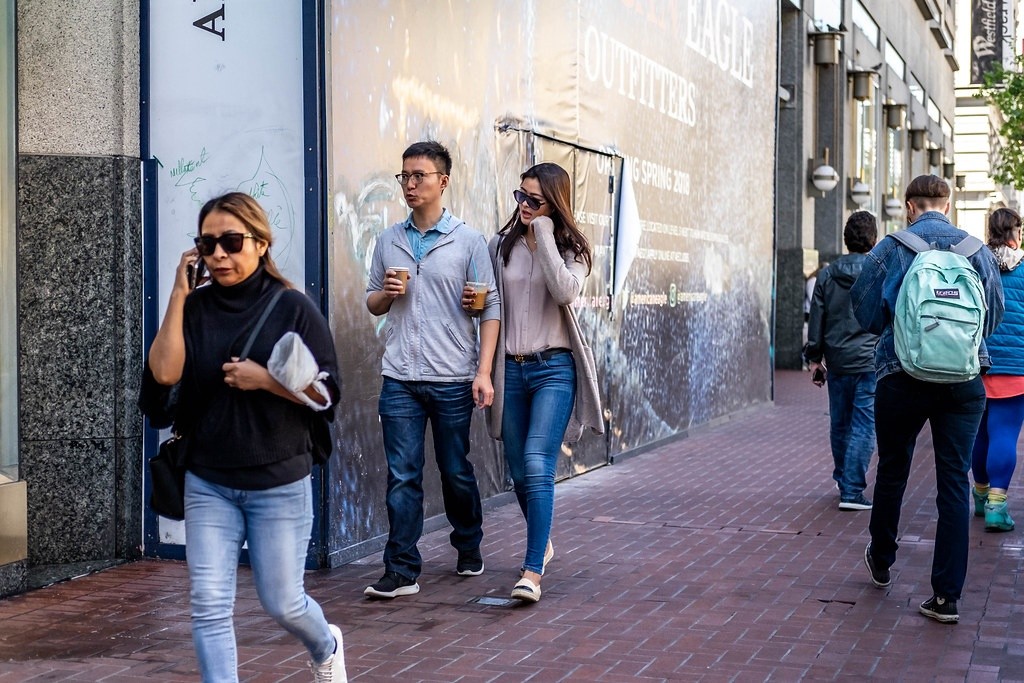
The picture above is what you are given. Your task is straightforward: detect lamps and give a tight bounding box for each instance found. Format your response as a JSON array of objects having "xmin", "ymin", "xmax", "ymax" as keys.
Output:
[
  {"xmin": 848, "ymin": 67, "xmax": 879, "ymax": 100},
  {"xmin": 808, "ymin": 29, "xmax": 841, "ymax": 63},
  {"xmin": 956, "ymin": 175, "xmax": 966, "ymax": 190},
  {"xmin": 908, "ymin": 128, "xmax": 930, "ymax": 152},
  {"xmin": 927, "ymin": 147, "xmax": 944, "ymax": 166},
  {"xmin": 811, "ymin": 165, "xmax": 902, "ymax": 217},
  {"xmin": 943, "ymin": 163, "xmax": 956, "ymax": 180},
  {"xmin": 884, "ymin": 103, "xmax": 909, "ymax": 129}
]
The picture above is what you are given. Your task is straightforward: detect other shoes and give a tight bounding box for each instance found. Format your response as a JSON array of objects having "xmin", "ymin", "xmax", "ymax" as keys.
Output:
[
  {"xmin": 521, "ymin": 539, "xmax": 554, "ymax": 577},
  {"xmin": 511, "ymin": 579, "xmax": 542, "ymax": 603}
]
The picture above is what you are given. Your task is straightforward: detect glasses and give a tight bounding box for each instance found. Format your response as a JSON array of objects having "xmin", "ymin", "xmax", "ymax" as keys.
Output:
[
  {"xmin": 513, "ymin": 190, "xmax": 545, "ymax": 211},
  {"xmin": 394, "ymin": 171, "xmax": 444, "ymax": 187},
  {"xmin": 194, "ymin": 232, "xmax": 253, "ymax": 256}
]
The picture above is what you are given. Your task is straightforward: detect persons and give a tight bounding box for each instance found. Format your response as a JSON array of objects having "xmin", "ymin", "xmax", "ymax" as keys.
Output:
[
  {"xmin": 849, "ymin": 174, "xmax": 1005, "ymax": 622},
  {"xmin": 461, "ymin": 162, "xmax": 605, "ymax": 602},
  {"xmin": 971, "ymin": 208, "xmax": 1024, "ymax": 529},
  {"xmin": 803, "ymin": 262, "xmax": 830, "ymax": 371},
  {"xmin": 804, "ymin": 211, "xmax": 877, "ymax": 509},
  {"xmin": 365, "ymin": 139, "xmax": 500, "ymax": 597},
  {"xmin": 138, "ymin": 192, "xmax": 348, "ymax": 683}
]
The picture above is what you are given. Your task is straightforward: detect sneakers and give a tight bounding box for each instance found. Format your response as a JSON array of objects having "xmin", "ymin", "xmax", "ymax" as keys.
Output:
[
  {"xmin": 839, "ymin": 495, "xmax": 873, "ymax": 510},
  {"xmin": 918, "ymin": 594, "xmax": 960, "ymax": 621},
  {"xmin": 455, "ymin": 557, "xmax": 485, "ymax": 576},
  {"xmin": 306, "ymin": 623, "xmax": 348, "ymax": 683},
  {"xmin": 984, "ymin": 498, "xmax": 1016, "ymax": 532},
  {"xmin": 363, "ymin": 573, "xmax": 419, "ymax": 597},
  {"xmin": 973, "ymin": 487, "xmax": 990, "ymax": 516},
  {"xmin": 864, "ymin": 541, "xmax": 891, "ymax": 587}
]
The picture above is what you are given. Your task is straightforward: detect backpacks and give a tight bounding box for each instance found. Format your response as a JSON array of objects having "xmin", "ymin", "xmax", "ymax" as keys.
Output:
[{"xmin": 887, "ymin": 231, "xmax": 987, "ymax": 385}]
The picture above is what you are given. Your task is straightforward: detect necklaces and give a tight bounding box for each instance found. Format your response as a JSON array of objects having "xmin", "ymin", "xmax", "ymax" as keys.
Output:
[{"xmin": 525, "ymin": 234, "xmax": 537, "ymax": 243}]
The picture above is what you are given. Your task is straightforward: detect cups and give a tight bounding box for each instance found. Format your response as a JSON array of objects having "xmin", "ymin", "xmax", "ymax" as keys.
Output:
[
  {"xmin": 389, "ymin": 267, "xmax": 409, "ymax": 295},
  {"xmin": 465, "ymin": 282, "xmax": 490, "ymax": 309}
]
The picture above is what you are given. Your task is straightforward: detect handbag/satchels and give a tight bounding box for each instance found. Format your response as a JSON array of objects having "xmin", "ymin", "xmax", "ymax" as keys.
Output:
[
  {"xmin": 267, "ymin": 332, "xmax": 332, "ymax": 411},
  {"xmin": 147, "ymin": 437, "xmax": 186, "ymax": 522}
]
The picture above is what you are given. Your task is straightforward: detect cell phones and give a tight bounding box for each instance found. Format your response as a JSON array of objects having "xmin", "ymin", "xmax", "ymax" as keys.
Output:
[
  {"xmin": 191, "ymin": 257, "xmax": 206, "ymax": 288},
  {"xmin": 813, "ymin": 369, "xmax": 825, "ymax": 386}
]
